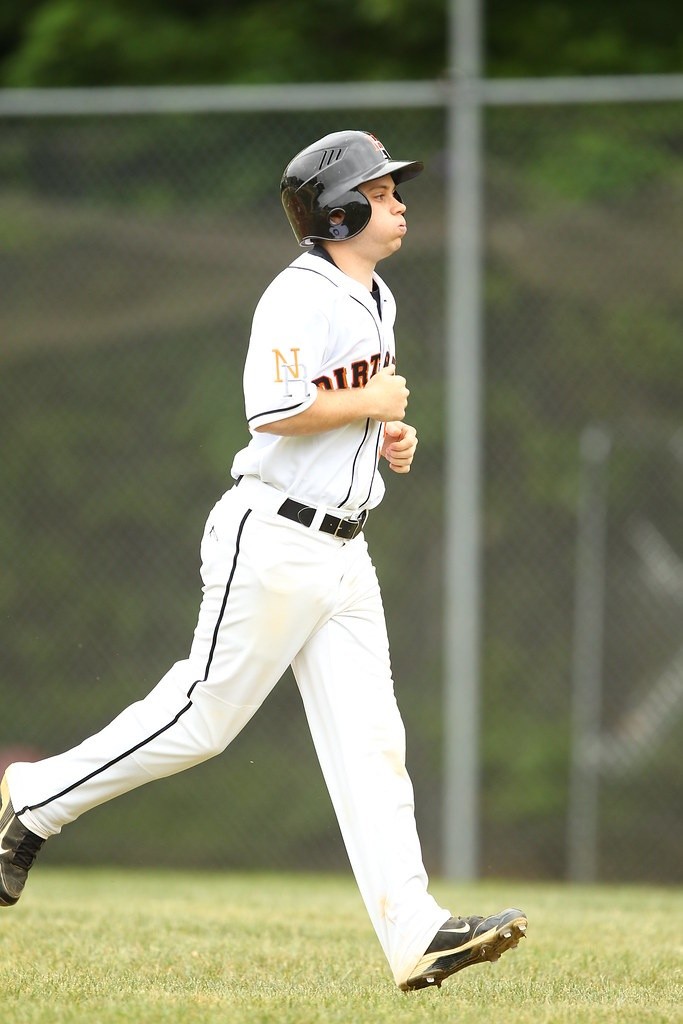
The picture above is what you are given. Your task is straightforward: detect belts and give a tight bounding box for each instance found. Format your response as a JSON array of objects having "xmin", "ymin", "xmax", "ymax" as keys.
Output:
[{"xmin": 278, "ymin": 498, "xmax": 370, "ymax": 539}]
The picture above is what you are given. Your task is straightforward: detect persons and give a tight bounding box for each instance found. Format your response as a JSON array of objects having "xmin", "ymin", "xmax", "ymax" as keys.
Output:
[{"xmin": 0, "ymin": 130, "xmax": 526, "ymax": 993}]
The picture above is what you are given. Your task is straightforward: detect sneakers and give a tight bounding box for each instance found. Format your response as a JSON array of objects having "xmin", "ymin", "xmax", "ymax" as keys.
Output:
[
  {"xmin": 0, "ymin": 774, "xmax": 48, "ymax": 906},
  {"xmin": 408, "ymin": 907, "xmax": 529, "ymax": 990}
]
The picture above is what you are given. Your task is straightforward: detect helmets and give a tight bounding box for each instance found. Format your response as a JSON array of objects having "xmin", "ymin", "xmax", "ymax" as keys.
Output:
[{"xmin": 278, "ymin": 130, "xmax": 425, "ymax": 247}]
